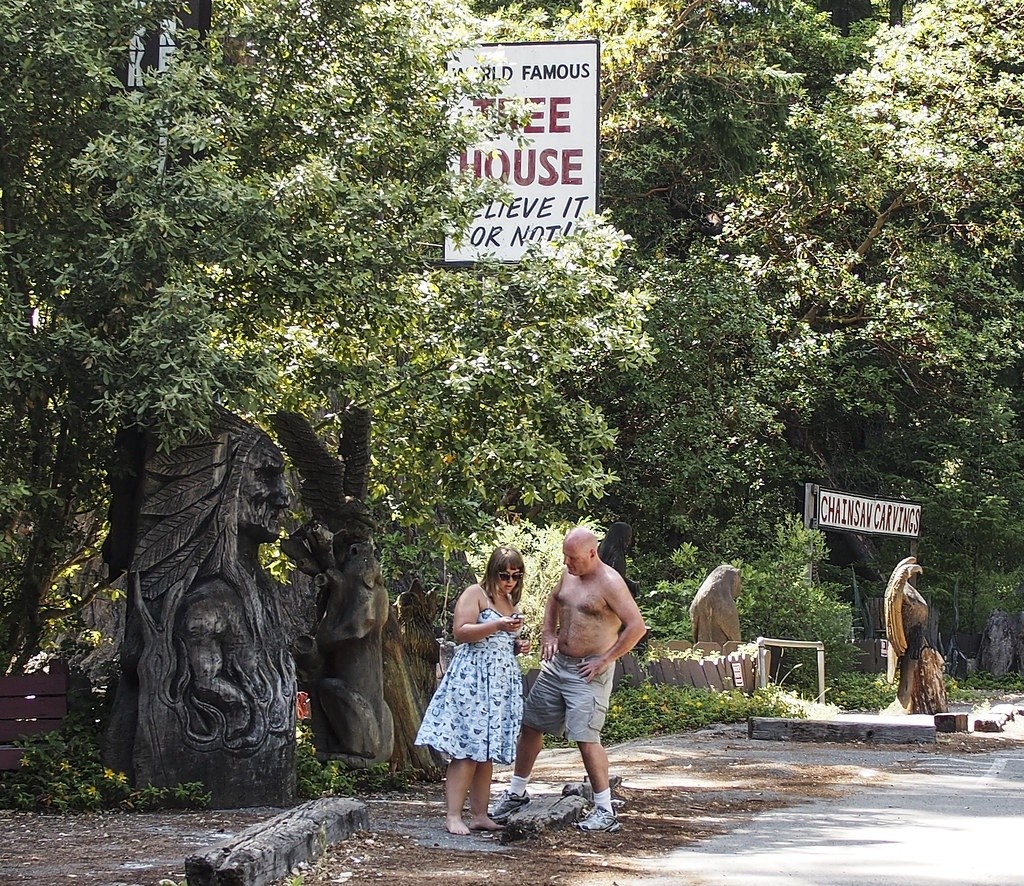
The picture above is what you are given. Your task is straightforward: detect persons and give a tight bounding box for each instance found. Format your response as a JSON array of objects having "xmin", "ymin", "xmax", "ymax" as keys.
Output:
[
  {"xmin": 487, "ymin": 527, "xmax": 647, "ymax": 832},
  {"xmin": 414, "ymin": 545, "xmax": 530, "ymax": 835},
  {"xmin": 597, "ymin": 522, "xmax": 641, "ymax": 634},
  {"xmin": 135, "ymin": 400, "xmax": 298, "ymax": 808}
]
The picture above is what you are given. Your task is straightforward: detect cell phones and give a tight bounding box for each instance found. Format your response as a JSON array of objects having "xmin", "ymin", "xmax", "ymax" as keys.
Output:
[{"xmin": 512, "ymin": 614, "xmax": 525, "ymax": 627}]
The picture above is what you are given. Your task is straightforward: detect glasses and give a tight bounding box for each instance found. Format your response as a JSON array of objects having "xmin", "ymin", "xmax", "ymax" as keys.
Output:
[{"xmin": 498, "ymin": 571, "xmax": 524, "ymax": 582}]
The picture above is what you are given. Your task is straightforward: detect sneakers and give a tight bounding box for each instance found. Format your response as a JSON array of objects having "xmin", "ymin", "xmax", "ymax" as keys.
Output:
[
  {"xmin": 486, "ymin": 789, "xmax": 532, "ymax": 820},
  {"xmin": 576, "ymin": 805, "xmax": 621, "ymax": 833}
]
